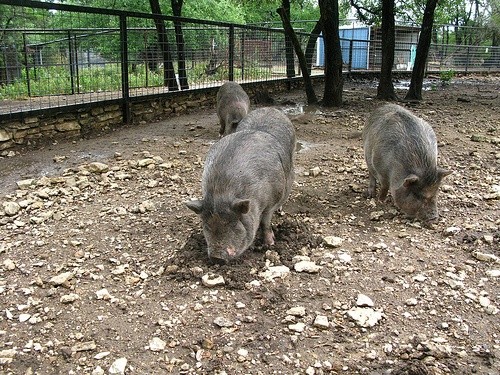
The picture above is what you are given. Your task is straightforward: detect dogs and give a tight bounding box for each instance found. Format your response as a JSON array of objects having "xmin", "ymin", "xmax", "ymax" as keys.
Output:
[{"xmin": 362, "ymin": 103, "xmax": 454, "ymax": 226}]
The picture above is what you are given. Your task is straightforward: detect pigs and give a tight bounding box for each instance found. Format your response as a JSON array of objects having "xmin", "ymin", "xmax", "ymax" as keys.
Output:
[
  {"xmin": 183, "ymin": 107, "xmax": 297, "ymax": 266},
  {"xmin": 216, "ymin": 82, "xmax": 251, "ymax": 138}
]
